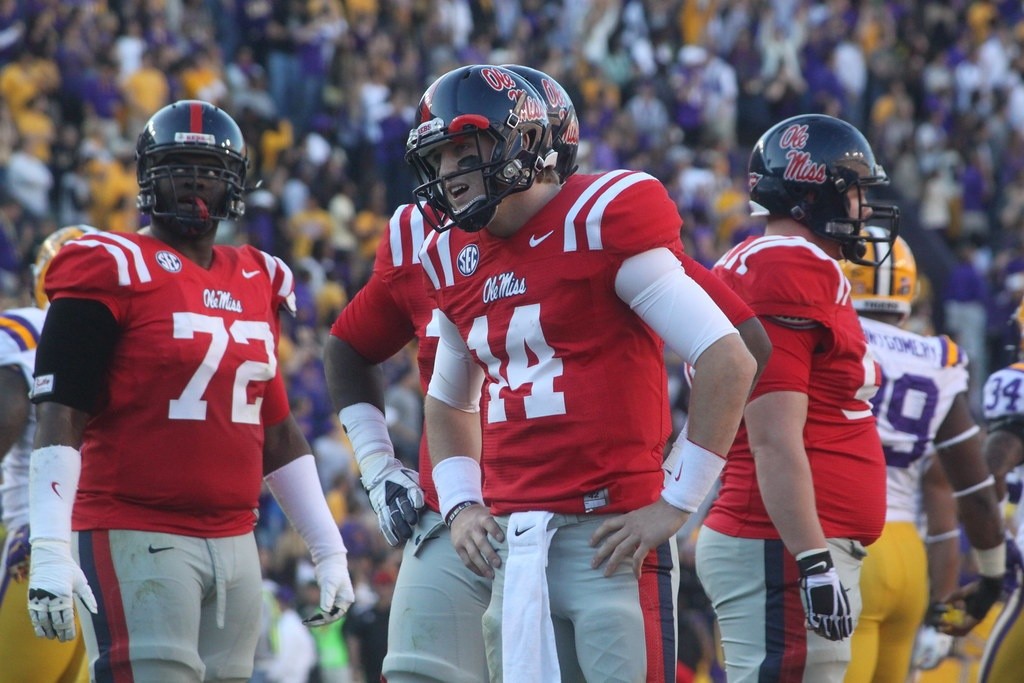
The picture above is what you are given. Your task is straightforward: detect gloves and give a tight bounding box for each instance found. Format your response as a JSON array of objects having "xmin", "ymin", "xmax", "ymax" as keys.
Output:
[
  {"xmin": 302, "ymin": 553, "xmax": 355, "ymax": 627},
  {"xmin": 360, "ymin": 455, "xmax": 427, "ymax": 548},
  {"xmin": 794, "ymin": 547, "xmax": 854, "ymax": 641},
  {"xmin": 26, "ymin": 540, "xmax": 98, "ymax": 642}
]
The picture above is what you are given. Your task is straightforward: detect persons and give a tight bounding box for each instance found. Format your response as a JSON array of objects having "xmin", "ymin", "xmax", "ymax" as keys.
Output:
[
  {"xmin": 0, "ymin": 1, "xmax": 1024, "ymax": 683},
  {"xmin": 27, "ymin": 98, "xmax": 355, "ymax": 683}
]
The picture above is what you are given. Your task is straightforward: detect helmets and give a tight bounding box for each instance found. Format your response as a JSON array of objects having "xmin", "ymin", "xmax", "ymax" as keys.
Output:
[
  {"xmin": 404, "ymin": 64, "xmax": 558, "ymax": 235},
  {"xmin": 837, "ymin": 225, "xmax": 917, "ymax": 323},
  {"xmin": 30, "ymin": 225, "xmax": 98, "ymax": 309},
  {"xmin": 748, "ymin": 114, "xmax": 900, "ymax": 269},
  {"xmin": 502, "ymin": 64, "xmax": 580, "ymax": 183},
  {"xmin": 1004, "ymin": 301, "xmax": 1024, "ymax": 370},
  {"xmin": 135, "ymin": 100, "xmax": 249, "ymax": 241}
]
